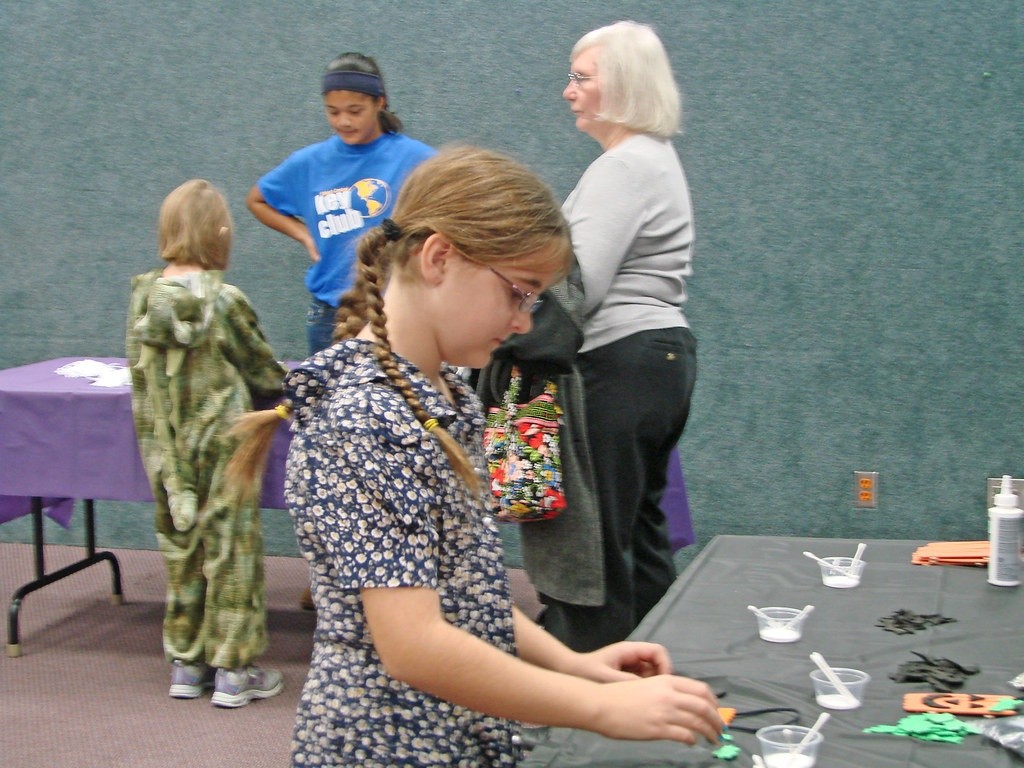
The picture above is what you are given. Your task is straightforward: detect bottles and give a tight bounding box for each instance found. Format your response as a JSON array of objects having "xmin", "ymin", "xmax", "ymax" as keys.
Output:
[{"xmin": 987, "ymin": 475, "xmax": 1024, "ymax": 587}]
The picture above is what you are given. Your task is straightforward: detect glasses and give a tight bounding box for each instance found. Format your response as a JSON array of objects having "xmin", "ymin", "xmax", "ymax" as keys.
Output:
[
  {"xmin": 568, "ymin": 72, "xmax": 604, "ymax": 87},
  {"xmin": 482, "ymin": 261, "xmax": 545, "ymax": 315}
]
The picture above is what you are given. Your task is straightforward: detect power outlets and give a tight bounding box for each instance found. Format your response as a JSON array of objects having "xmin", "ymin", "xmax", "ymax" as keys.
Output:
[{"xmin": 854, "ymin": 471, "xmax": 877, "ymax": 508}]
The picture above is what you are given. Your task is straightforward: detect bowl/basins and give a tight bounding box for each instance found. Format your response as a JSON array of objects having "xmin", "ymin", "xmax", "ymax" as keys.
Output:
[
  {"xmin": 756, "ymin": 725, "xmax": 824, "ymax": 768},
  {"xmin": 810, "ymin": 668, "xmax": 870, "ymax": 709},
  {"xmin": 755, "ymin": 607, "xmax": 807, "ymax": 642},
  {"xmin": 818, "ymin": 557, "xmax": 865, "ymax": 589}
]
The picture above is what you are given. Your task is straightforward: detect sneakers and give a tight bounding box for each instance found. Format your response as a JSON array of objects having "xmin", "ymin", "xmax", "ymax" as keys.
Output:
[
  {"xmin": 170, "ymin": 659, "xmax": 217, "ymax": 699},
  {"xmin": 211, "ymin": 664, "xmax": 284, "ymax": 707}
]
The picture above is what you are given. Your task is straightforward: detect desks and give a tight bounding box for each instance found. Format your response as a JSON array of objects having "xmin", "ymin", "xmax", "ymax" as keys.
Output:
[
  {"xmin": 0, "ymin": 357, "xmax": 694, "ymax": 658},
  {"xmin": 520, "ymin": 535, "xmax": 1024, "ymax": 767}
]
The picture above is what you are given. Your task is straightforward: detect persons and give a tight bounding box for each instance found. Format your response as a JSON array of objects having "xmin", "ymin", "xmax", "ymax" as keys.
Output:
[
  {"xmin": 124, "ymin": 179, "xmax": 290, "ymax": 707},
  {"xmin": 288, "ymin": 142, "xmax": 723, "ymax": 768},
  {"xmin": 483, "ymin": 22, "xmax": 695, "ymax": 654},
  {"xmin": 245, "ymin": 51, "xmax": 436, "ymax": 356}
]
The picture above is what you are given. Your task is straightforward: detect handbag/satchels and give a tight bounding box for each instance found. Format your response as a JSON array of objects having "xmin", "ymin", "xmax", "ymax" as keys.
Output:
[{"xmin": 483, "ymin": 360, "xmax": 565, "ymax": 523}]
